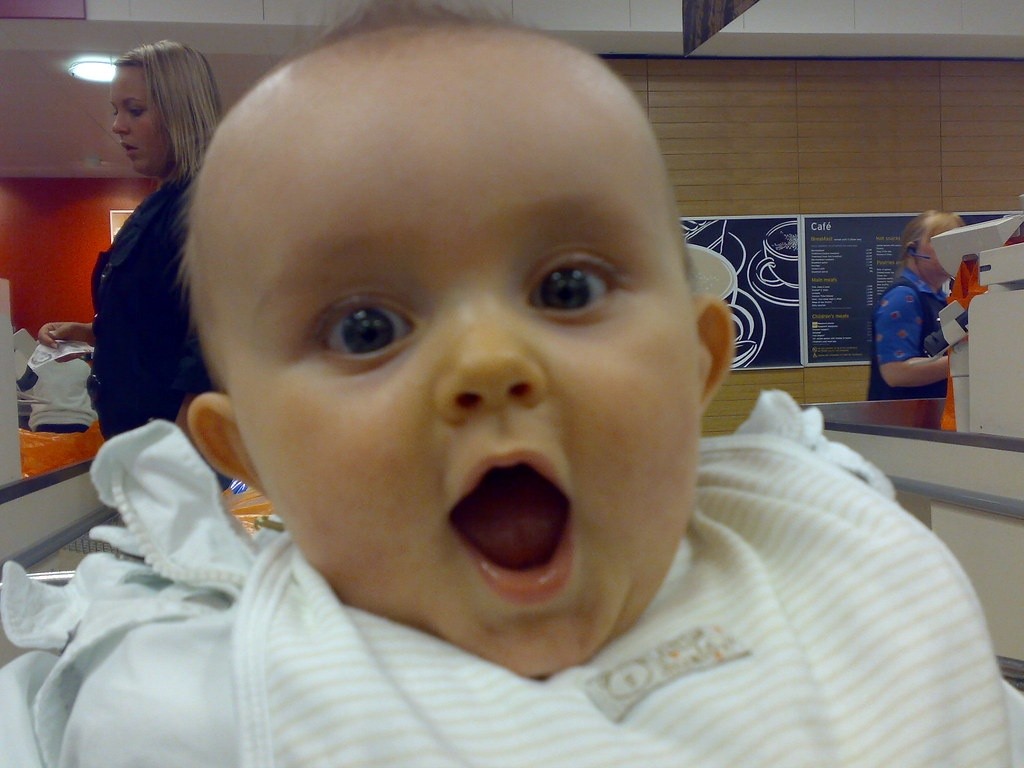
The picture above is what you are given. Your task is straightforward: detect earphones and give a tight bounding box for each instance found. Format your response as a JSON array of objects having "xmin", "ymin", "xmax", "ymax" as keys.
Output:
[{"xmin": 907, "ymin": 246, "xmax": 915, "ymax": 254}]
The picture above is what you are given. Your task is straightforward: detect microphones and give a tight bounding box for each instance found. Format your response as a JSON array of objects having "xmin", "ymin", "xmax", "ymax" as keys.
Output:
[{"xmin": 913, "ymin": 253, "xmax": 930, "ymax": 259}]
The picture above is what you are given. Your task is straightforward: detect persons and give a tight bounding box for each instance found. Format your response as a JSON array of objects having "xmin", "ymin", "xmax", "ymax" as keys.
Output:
[
  {"xmin": 1, "ymin": 0, "xmax": 1024, "ymax": 768},
  {"xmin": 869, "ymin": 205, "xmax": 966, "ymax": 403},
  {"xmin": 38, "ymin": 41, "xmax": 226, "ymax": 444}
]
